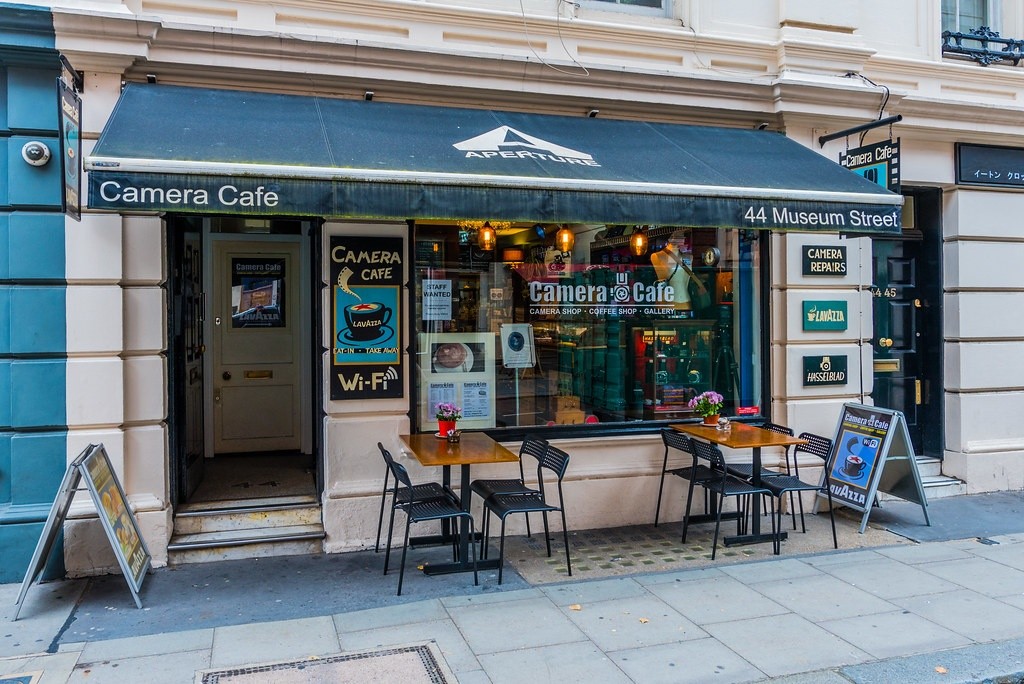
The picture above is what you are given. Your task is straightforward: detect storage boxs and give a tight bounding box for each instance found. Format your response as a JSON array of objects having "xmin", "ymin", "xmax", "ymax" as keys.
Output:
[
  {"xmin": 550, "ymin": 409, "xmax": 585, "ymax": 424},
  {"xmin": 552, "ymin": 396, "xmax": 581, "ymax": 412}
]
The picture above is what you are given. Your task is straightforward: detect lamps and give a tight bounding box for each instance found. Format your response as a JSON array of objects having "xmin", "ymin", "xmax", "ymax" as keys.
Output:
[
  {"xmin": 555, "ymin": 224, "xmax": 575, "ymax": 252},
  {"xmin": 629, "ymin": 225, "xmax": 649, "ymax": 255},
  {"xmin": 477, "ymin": 221, "xmax": 497, "ymax": 251}
]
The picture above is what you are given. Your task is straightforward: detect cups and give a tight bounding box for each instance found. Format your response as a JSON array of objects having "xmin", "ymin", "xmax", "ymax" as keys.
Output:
[{"xmin": 344, "ymin": 301, "xmax": 393, "ymax": 338}]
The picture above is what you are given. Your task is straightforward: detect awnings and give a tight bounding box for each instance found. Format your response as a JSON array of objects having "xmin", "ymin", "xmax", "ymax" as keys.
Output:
[{"xmin": 83, "ymin": 81, "xmax": 907, "ymax": 235}]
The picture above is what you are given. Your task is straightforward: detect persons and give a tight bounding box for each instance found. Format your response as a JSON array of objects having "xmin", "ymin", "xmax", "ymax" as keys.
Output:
[{"xmin": 650, "ymin": 236, "xmax": 694, "ymax": 313}]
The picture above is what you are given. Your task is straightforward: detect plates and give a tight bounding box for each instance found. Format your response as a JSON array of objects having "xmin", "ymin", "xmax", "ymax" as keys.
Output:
[
  {"xmin": 337, "ymin": 325, "xmax": 394, "ymax": 346},
  {"xmin": 432, "ymin": 344, "xmax": 474, "ymax": 373}
]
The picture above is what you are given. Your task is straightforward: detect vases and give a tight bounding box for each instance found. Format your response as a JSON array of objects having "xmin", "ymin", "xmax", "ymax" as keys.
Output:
[
  {"xmin": 438, "ymin": 420, "xmax": 456, "ymax": 436},
  {"xmin": 703, "ymin": 414, "xmax": 720, "ymax": 424}
]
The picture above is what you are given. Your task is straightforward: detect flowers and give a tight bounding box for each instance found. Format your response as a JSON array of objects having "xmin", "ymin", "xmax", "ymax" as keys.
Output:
[
  {"xmin": 683, "ymin": 388, "xmax": 698, "ymax": 402},
  {"xmin": 689, "ymin": 390, "xmax": 724, "ymax": 416},
  {"xmin": 435, "ymin": 400, "xmax": 463, "ymax": 421}
]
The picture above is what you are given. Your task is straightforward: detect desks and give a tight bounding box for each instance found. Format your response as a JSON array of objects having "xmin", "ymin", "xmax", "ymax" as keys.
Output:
[
  {"xmin": 669, "ymin": 421, "xmax": 809, "ymax": 547},
  {"xmin": 399, "ymin": 432, "xmax": 520, "ymax": 574}
]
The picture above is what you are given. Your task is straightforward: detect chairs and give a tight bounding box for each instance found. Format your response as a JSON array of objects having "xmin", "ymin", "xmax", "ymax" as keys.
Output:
[
  {"xmin": 376, "ymin": 442, "xmax": 460, "ymax": 569},
  {"xmin": 469, "ymin": 433, "xmax": 549, "ymax": 560},
  {"xmin": 383, "ymin": 453, "xmax": 479, "ymax": 596},
  {"xmin": 485, "ymin": 446, "xmax": 573, "ymax": 586},
  {"xmin": 652, "ymin": 420, "xmax": 838, "ymax": 560}
]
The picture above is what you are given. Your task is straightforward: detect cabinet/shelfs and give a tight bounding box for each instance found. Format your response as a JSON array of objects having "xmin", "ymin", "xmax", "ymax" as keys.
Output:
[
  {"xmin": 589, "ymin": 227, "xmax": 717, "ymax": 422},
  {"xmin": 624, "ymin": 318, "xmax": 718, "ymax": 420}
]
